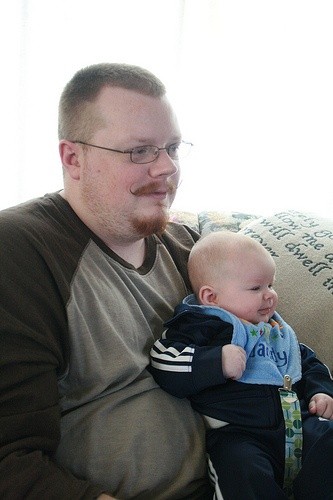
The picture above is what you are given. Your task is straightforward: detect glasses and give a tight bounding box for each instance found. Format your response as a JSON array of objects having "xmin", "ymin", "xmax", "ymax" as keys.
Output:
[{"xmin": 70, "ymin": 140, "xmax": 194, "ymax": 165}]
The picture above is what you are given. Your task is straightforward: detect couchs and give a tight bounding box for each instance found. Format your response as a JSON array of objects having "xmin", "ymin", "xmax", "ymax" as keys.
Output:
[{"xmin": 165, "ymin": 212, "xmax": 333, "ymax": 379}]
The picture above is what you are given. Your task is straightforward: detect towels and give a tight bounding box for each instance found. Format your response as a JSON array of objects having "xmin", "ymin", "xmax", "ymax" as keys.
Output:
[{"xmin": 182, "ymin": 293, "xmax": 303, "ymax": 386}]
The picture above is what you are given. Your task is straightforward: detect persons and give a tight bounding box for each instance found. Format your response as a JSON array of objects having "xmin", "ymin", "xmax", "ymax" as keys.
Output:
[
  {"xmin": 0, "ymin": 62, "xmax": 218, "ymax": 500},
  {"xmin": 149, "ymin": 231, "xmax": 333, "ymax": 500}
]
